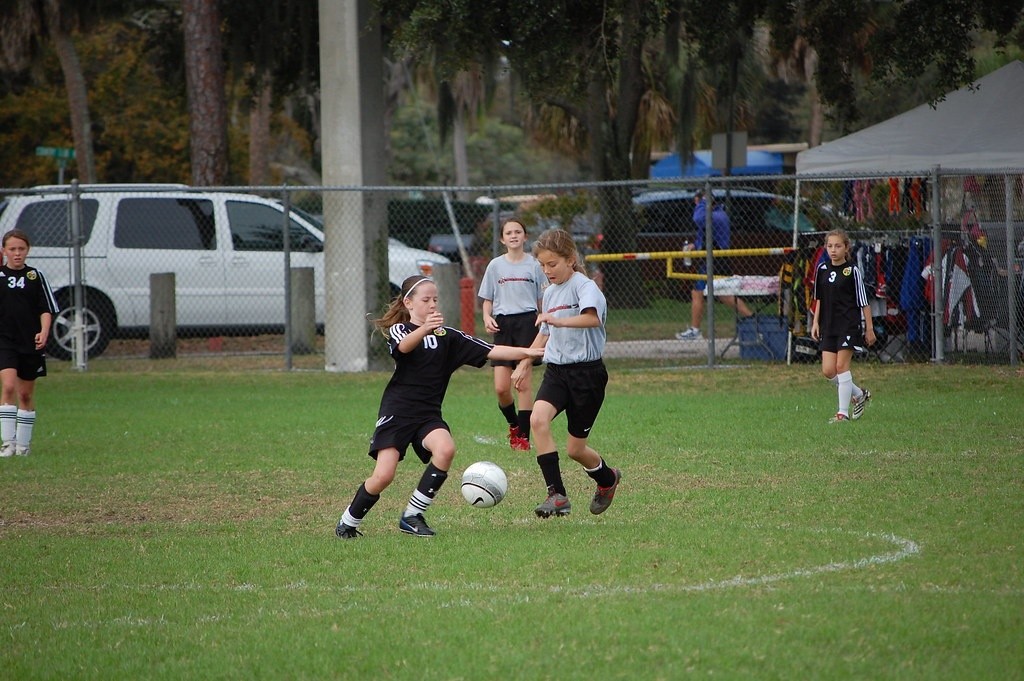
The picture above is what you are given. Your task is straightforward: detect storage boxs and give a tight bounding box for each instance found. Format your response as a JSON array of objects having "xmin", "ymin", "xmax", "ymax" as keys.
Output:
[{"xmin": 739, "ymin": 314, "xmax": 788, "ymax": 359}]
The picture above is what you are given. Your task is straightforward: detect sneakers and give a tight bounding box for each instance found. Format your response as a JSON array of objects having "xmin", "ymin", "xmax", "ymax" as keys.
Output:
[
  {"xmin": 535, "ymin": 488, "xmax": 572, "ymax": 518},
  {"xmin": 399, "ymin": 512, "xmax": 436, "ymax": 538},
  {"xmin": 16, "ymin": 445, "xmax": 32, "ymax": 457},
  {"xmin": 513, "ymin": 436, "xmax": 530, "ymax": 450},
  {"xmin": 335, "ymin": 518, "xmax": 363, "ymax": 539},
  {"xmin": 851, "ymin": 388, "xmax": 871, "ymax": 420},
  {"xmin": 829, "ymin": 413, "xmax": 850, "ymax": 424},
  {"xmin": 676, "ymin": 328, "xmax": 703, "ymax": 339},
  {"xmin": 589, "ymin": 467, "xmax": 622, "ymax": 515},
  {"xmin": 508, "ymin": 424, "xmax": 520, "ymax": 451},
  {"xmin": 0, "ymin": 440, "xmax": 16, "ymax": 457}
]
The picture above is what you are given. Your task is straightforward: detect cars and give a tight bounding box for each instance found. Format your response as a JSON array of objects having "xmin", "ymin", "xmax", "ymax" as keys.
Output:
[{"xmin": 304, "ymin": 186, "xmax": 872, "ymax": 308}]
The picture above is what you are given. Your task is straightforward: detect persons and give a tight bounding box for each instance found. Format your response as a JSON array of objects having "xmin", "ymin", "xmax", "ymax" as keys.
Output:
[
  {"xmin": 336, "ymin": 275, "xmax": 545, "ymax": 539},
  {"xmin": 811, "ymin": 229, "xmax": 876, "ymax": 424},
  {"xmin": 0, "ymin": 230, "xmax": 59, "ymax": 457},
  {"xmin": 675, "ymin": 190, "xmax": 751, "ymax": 340},
  {"xmin": 510, "ymin": 229, "xmax": 622, "ymax": 519},
  {"xmin": 477, "ymin": 217, "xmax": 550, "ymax": 451}
]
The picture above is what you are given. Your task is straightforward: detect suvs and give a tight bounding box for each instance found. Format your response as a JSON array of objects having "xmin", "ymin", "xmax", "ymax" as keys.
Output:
[{"xmin": 0, "ymin": 183, "xmax": 452, "ymax": 361}]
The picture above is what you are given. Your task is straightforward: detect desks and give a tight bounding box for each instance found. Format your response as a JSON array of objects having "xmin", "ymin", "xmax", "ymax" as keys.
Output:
[{"xmin": 703, "ymin": 288, "xmax": 777, "ymax": 362}]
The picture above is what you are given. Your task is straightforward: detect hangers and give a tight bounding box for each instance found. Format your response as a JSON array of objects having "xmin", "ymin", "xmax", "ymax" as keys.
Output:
[{"xmin": 854, "ymin": 227, "xmax": 974, "ymax": 253}]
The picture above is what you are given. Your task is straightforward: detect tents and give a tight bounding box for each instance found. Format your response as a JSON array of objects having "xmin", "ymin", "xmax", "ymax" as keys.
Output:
[{"xmin": 791, "ymin": 59, "xmax": 1024, "ymax": 249}]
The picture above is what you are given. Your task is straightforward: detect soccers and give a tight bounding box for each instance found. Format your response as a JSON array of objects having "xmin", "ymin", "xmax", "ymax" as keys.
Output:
[{"xmin": 460, "ymin": 461, "xmax": 508, "ymax": 508}]
